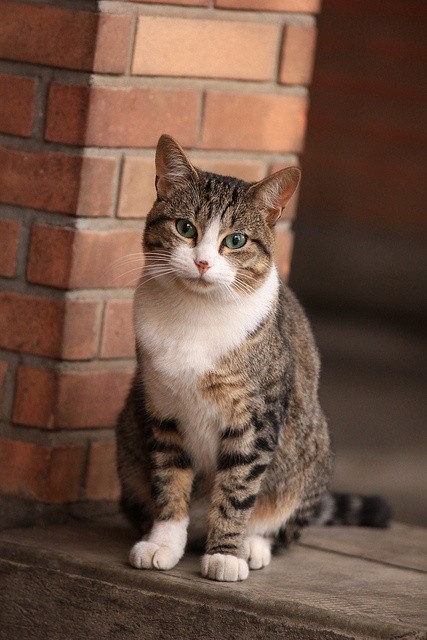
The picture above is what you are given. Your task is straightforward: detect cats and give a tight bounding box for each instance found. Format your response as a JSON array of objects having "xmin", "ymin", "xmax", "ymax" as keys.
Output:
[{"xmin": 114, "ymin": 133, "xmax": 393, "ymax": 582}]
